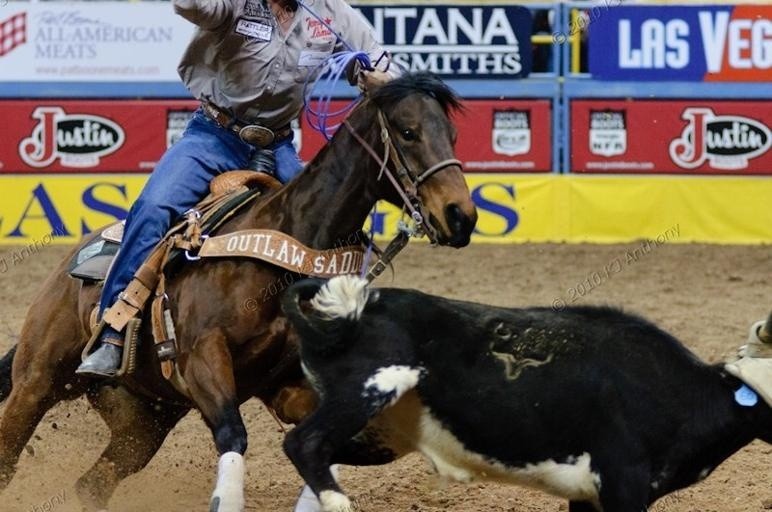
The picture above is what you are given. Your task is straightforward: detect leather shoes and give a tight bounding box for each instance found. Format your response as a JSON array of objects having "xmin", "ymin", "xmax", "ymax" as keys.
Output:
[{"xmin": 74, "ymin": 342, "xmax": 123, "ymax": 383}]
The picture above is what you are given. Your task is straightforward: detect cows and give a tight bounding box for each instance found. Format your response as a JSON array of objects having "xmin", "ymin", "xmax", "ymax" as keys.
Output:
[{"xmin": 279, "ymin": 272, "xmax": 771, "ymax": 511}]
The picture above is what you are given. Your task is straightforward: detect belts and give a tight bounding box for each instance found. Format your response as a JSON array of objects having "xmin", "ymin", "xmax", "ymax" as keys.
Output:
[{"xmin": 199, "ymin": 101, "xmax": 291, "ymax": 148}]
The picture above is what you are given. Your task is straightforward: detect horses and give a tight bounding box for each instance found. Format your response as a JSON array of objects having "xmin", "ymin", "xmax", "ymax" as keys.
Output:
[{"xmin": 0, "ymin": 71, "xmax": 478, "ymax": 511}]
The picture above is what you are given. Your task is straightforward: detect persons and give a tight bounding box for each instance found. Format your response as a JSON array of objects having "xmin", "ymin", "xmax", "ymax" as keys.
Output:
[{"xmin": 76, "ymin": 0, "xmax": 408, "ymax": 381}]
